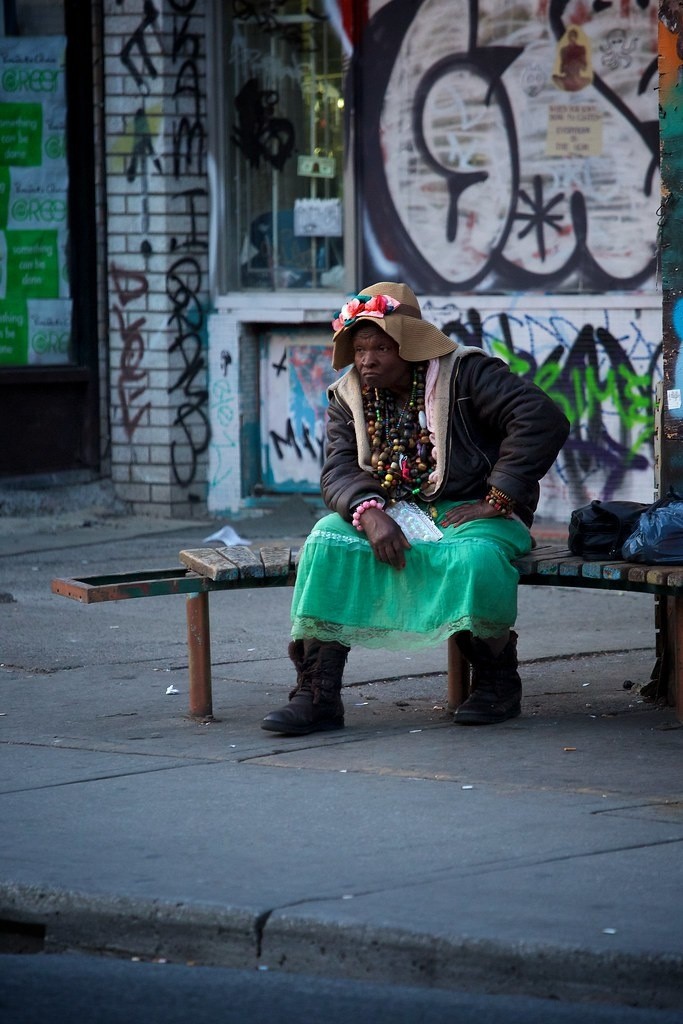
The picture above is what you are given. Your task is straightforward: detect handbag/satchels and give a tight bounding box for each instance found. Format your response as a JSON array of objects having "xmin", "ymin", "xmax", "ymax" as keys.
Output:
[
  {"xmin": 568, "ymin": 485, "xmax": 681, "ymax": 561},
  {"xmin": 621, "ymin": 501, "xmax": 683, "ymax": 563}
]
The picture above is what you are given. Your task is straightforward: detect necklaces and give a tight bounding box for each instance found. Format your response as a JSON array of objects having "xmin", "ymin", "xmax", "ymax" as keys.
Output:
[{"xmin": 359, "ymin": 362, "xmax": 435, "ymax": 506}]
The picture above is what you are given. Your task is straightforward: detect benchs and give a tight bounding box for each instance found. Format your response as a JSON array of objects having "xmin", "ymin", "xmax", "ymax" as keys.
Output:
[{"xmin": 51, "ymin": 541, "xmax": 683, "ymax": 713}]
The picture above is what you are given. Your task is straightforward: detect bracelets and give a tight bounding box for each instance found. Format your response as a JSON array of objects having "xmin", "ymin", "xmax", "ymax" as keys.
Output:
[
  {"xmin": 353, "ymin": 500, "xmax": 383, "ymax": 531},
  {"xmin": 485, "ymin": 487, "xmax": 514, "ymax": 515}
]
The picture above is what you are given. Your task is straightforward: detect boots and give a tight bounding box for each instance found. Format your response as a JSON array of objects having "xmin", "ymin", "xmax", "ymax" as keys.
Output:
[
  {"xmin": 260, "ymin": 639, "xmax": 350, "ymax": 734},
  {"xmin": 454, "ymin": 630, "xmax": 522, "ymax": 723}
]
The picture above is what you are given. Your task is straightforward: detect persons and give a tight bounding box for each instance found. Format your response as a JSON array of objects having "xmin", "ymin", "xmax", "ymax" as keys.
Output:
[
  {"xmin": 268, "ymin": 211, "xmax": 339, "ymax": 288},
  {"xmin": 261, "ymin": 282, "xmax": 569, "ymax": 731}
]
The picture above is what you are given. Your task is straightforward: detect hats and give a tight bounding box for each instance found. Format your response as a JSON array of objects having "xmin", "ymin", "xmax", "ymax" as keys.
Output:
[{"xmin": 332, "ymin": 282, "xmax": 459, "ymax": 372}]
